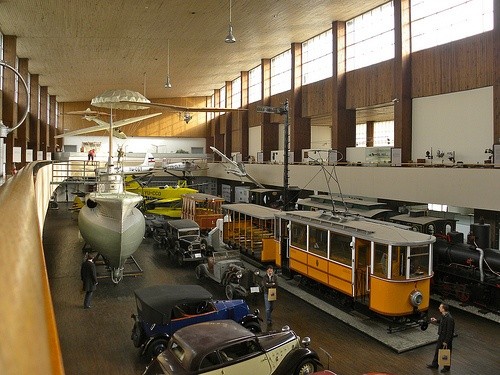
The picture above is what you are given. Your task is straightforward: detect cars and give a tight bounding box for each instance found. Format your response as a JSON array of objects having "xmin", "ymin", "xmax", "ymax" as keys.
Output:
[
  {"xmin": 131, "ymin": 285, "xmax": 339, "ymax": 375},
  {"xmin": 143, "ymin": 212, "xmax": 266, "ymax": 300}
]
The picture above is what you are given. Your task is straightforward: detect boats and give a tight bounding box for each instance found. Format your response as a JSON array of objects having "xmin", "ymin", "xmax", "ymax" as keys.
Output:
[{"xmin": 78, "ymin": 89, "xmax": 147, "ymax": 284}]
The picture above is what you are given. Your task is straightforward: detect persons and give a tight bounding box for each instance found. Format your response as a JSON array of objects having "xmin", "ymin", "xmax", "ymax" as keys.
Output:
[
  {"xmin": 86, "ymin": 148, "xmax": 95, "ymax": 165},
  {"xmin": 426, "ymin": 302, "xmax": 454, "ymax": 372},
  {"xmin": 81, "ymin": 254, "xmax": 98, "ymax": 309},
  {"xmin": 263, "ymin": 264, "xmax": 279, "ymax": 326}
]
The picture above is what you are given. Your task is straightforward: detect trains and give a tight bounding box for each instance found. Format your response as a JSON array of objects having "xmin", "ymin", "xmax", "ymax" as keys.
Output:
[
  {"xmin": 182, "ymin": 194, "xmax": 439, "ymax": 334},
  {"xmin": 295, "ymin": 193, "xmax": 500, "ymax": 318}
]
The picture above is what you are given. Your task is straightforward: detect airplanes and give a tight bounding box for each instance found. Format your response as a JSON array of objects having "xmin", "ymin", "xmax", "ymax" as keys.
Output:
[
  {"xmin": 94, "ymin": 152, "xmax": 155, "ymax": 175},
  {"xmin": 210, "ymin": 146, "xmax": 265, "ymax": 189},
  {"xmin": 162, "ymin": 157, "xmax": 205, "ymax": 172},
  {"xmin": 124, "ymin": 177, "xmax": 199, "ymax": 203},
  {"xmin": 54, "ymin": 108, "xmax": 162, "ymax": 139}
]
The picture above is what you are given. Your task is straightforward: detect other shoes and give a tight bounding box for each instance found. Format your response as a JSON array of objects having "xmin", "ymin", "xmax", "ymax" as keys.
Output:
[
  {"xmin": 84, "ymin": 306, "xmax": 92, "ymax": 309},
  {"xmin": 267, "ymin": 320, "xmax": 271, "ymax": 324}
]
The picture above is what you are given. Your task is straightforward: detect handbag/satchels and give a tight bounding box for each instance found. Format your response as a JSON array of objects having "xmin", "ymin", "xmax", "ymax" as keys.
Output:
[
  {"xmin": 268, "ymin": 286, "xmax": 276, "ymax": 301},
  {"xmin": 438, "ymin": 345, "xmax": 450, "ymax": 366}
]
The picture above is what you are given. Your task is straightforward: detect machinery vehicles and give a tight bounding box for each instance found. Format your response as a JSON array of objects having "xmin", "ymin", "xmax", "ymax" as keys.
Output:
[{"xmin": 73, "ymin": 174, "xmax": 201, "ymax": 222}]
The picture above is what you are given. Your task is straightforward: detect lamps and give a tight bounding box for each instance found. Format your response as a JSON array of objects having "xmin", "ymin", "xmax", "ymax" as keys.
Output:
[
  {"xmin": 165, "ymin": 38, "xmax": 173, "ymax": 88},
  {"xmin": 225, "ymin": 0, "xmax": 236, "ymax": 42}
]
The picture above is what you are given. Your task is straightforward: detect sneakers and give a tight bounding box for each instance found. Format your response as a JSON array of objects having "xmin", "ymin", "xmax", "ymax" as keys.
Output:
[
  {"xmin": 427, "ymin": 364, "xmax": 438, "ymax": 369},
  {"xmin": 441, "ymin": 368, "xmax": 448, "ymax": 373}
]
